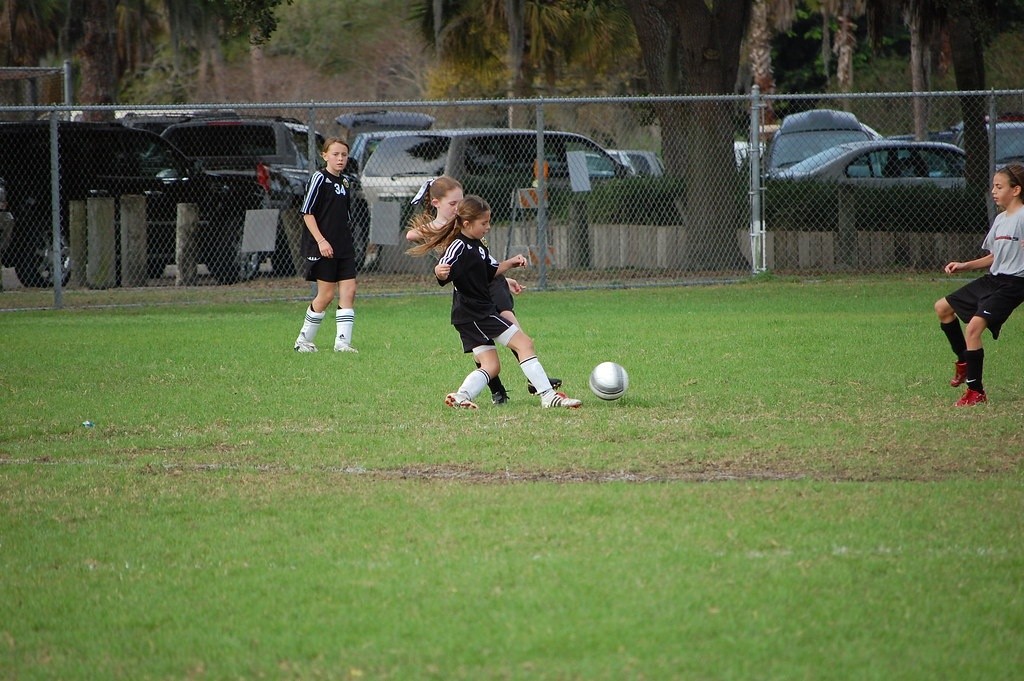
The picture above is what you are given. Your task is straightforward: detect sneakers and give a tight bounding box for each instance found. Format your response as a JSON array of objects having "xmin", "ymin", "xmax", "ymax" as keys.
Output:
[
  {"xmin": 955, "ymin": 388, "xmax": 986, "ymax": 408},
  {"xmin": 951, "ymin": 360, "xmax": 968, "ymax": 387},
  {"xmin": 491, "ymin": 389, "xmax": 512, "ymax": 404},
  {"xmin": 294, "ymin": 339, "xmax": 319, "ymax": 353},
  {"xmin": 540, "ymin": 391, "xmax": 582, "ymax": 410},
  {"xmin": 445, "ymin": 392, "xmax": 478, "ymax": 411},
  {"xmin": 528, "ymin": 378, "xmax": 562, "ymax": 394},
  {"xmin": 334, "ymin": 345, "xmax": 358, "ymax": 354}
]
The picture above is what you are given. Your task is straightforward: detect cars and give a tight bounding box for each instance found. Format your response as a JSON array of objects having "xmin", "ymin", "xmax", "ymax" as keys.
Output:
[{"xmin": 762, "ymin": 141, "xmax": 968, "ymax": 193}]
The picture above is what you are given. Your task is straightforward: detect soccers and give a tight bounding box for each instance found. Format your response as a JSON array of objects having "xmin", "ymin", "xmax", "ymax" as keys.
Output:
[{"xmin": 589, "ymin": 361, "xmax": 631, "ymax": 402}]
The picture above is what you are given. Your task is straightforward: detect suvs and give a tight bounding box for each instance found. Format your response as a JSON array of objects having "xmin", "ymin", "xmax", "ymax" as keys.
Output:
[
  {"xmin": 0, "ymin": 120, "xmax": 295, "ymax": 289},
  {"xmin": 582, "ymin": 150, "xmax": 666, "ymax": 178},
  {"xmin": 360, "ymin": 129, "xmax": 635, "ymax": 219},
  {"xmin": 273, "ymin": 118, "xmax": 329, "ymax": 168},
  {"xmin": 142, "ymin": 115, "xmax": 311, "ymax": 229},
  {"xmin": 730, "ymin": 107, "xmax": 1024, "ymax": 184}
]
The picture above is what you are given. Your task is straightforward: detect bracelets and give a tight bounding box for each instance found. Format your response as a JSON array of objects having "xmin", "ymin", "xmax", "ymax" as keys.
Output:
[{"xmin": 318, "ymin": 238, "xmax": 325, "ymax": 244}]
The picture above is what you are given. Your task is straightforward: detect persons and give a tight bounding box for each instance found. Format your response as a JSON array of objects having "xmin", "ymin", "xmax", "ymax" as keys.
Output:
[
  {"xmin": 294, "ymin": 137, "xmax": 359, "ymax": 354},
  {"xmin": 935, "ymin": 164, "xmax": 1024, "ymax": 408},
  {"xmin": 406, "ymin": 174, "xmax": 583, "ymax": 411}
]
